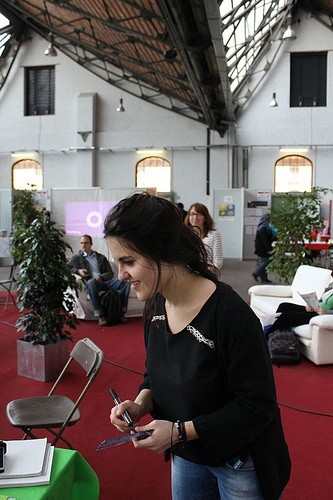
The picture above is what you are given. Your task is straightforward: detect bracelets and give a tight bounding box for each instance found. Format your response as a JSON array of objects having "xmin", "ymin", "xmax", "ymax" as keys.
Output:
[
  {"xmin": 171, "ymin": 421, "xmax": 174, "ymax": 451},
  {"xmin": 176, "ymin": 420, "xmax": 188, "ymax": 447}
]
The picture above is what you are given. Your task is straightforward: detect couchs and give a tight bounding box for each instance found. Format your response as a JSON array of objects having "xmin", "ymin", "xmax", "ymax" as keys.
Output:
[
  {"xmin": 248, "ymin": 264, "xmax": 333, "ymax": 366},
  {"xmin": 62, "ymin": 261, "xmax": 146, "ymax": 321}
]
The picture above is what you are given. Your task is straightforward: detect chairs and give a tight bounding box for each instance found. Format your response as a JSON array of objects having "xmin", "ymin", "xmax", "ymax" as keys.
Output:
[
  {"xmin": 6, "ymin": 337, "xmax": 104, "ymax": 450},
  {"xmin": 0, "ymin": 257, "xmax": 18, "ymax": 310}
]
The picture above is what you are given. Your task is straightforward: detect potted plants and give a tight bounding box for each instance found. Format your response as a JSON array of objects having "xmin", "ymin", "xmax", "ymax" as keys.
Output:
[{"xmin": 10, "ymin": 184, "xmax": 83, "ymax": 383}]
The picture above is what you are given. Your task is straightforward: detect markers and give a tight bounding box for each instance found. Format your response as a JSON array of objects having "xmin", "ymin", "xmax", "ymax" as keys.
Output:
[{"xmin": 106, "ymin": 385, "xmax": 139, "ymax": 435}]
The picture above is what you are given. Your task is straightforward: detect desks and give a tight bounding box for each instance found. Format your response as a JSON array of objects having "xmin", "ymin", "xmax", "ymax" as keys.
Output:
[{"xmin": 0, "ymin": 447, "xmax": 101, "ymax": 500}]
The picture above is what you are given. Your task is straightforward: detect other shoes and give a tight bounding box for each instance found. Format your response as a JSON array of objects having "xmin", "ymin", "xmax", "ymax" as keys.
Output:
[
  {"xmin": 99, "ymin": 317, "xmax": 106, "ymax": 325},
  {"xmin": 252, "ymin": 273, "xmax": 272, "ymax": 284},
  {"xmin": 120, "ymin": 317, "xmax": 129, "ymax": 323}
]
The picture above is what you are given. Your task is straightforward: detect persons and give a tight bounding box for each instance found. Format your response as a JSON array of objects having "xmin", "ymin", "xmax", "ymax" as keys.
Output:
[
  {"xmin": 175, "ymin": 203, "xmax": 188, "ymax": 221},
  {"xmin": 104, "ymin": 191, "xmax": 292, "ymax": 500},
  {"xmin": 252, "ymin": 213, "xmax": 279, "ymax": 283},
  {"xmin": 265, "ymin": 271, "xmax": 333, "ymax": 338},
  {"xmin": 184, "ymin": 203, "xmax": 223, "ymax": 275},
  {"xmin": 66, "ymin": 235, "xmax": 131, "ymax": 326}
]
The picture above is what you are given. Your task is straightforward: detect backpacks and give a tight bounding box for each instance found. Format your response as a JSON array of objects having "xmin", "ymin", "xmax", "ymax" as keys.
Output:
[{"xmin": 100, "ymin": 287, "xmax": 121, "ymax": 325}]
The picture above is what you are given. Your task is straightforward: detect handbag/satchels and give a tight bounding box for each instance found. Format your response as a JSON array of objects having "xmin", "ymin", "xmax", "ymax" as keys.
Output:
[{"xmin": 268, "ymin": 329, "xmax": 299, "ymax": 365}]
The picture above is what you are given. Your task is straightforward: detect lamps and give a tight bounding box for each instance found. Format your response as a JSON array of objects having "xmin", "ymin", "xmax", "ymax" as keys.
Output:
[
  {"xmin": 282, "ymin": 10, "xmax": 296, "ymax": 39},
  {"xmin": 44, "ymin": 31, "xmax": 57, "ymax": 56},
  {"xmin": 269, "ymin": 93, "xmax": 277, "ymax": 107},
  {"xmin": 117, "ymin": 98, "xmax": 125, "ymax": 112}
]
